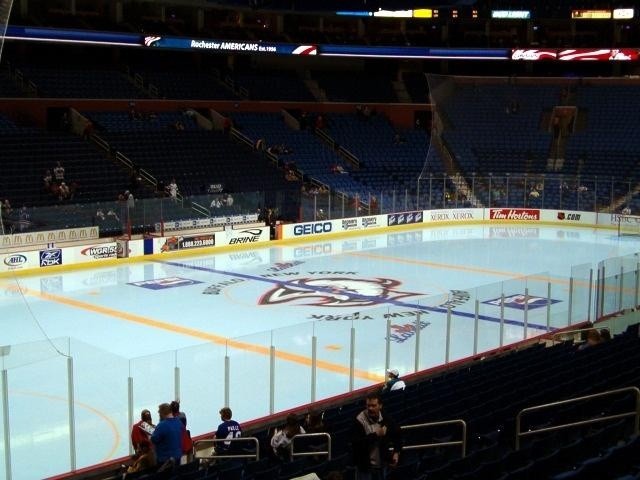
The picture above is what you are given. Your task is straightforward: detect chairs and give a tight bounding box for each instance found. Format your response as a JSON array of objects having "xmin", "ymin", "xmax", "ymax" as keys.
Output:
[
  {"xmin": 0, "ymin": 56, "xmax": 639, "ymax": 216},
  {"xmin": 80, "ymin": 322, "xmax": 640, "ymax": 479}
]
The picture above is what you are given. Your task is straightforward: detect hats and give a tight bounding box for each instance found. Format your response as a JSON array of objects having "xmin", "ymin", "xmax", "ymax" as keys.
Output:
[{"xmin": 387, "ymin": 368, "xmax": 399, "ymax": 375}]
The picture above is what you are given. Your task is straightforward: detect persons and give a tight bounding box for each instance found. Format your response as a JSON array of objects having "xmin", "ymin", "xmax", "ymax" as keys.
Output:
[
  {"xmin": 209, "ymin": 192, "xmax": 233, "ymax": 217},
  {"xmin": 151, "ymin": 404, "xmax": 183, "ymax": 465},
  {"xmin": 575, "ymin": 322, "xmax": 597, "ymax": 340},
  {"xmin": 384, "ymin": 365, "xmax": 408, "ymax": 393},
  {"xmin": 286, "ymin": 170, "xmax": 329, "ymax": 221},
  {"xmin": 368, "ymin": 196, "xmax": 378, "ymax": 216},
  {"xmin": 302, "ymin": 406, "xmax": 331, "ymax": 460},
  {"xmin": 578, "ymin": 328, "xmax": 603, "ymax": 352},
  {"xmin": 350, "ymin": 193, "xmax": 361, "ymax": 217},
  {"xmin": 491, "ymin": 185, "xmax": 506, "ymax": 199},
  {"xmin": 1, "ymin": 200, "xmax": 31, "ymax": 231},
  {"xmin": 560, "ymin": 182, "xmax": 587, "ymax": 191},
  {"xmin": 155, "ymin": 176, "xmax": 179, "ymax": 210},
  {"xmin": 96, "ymin": 190, "xmax": 135, "ymax": 226},
  {"xmin": 43, "ymin": 159, "xmax": 79, "ymax": 201},
  {"xmin": 599, "ymin": 325, "xmax": 612, "ymax": 342},
  {"xmin": 214, "ymin": 408, "xmax": 246, "ymax": 454},
  {"xmin": 621, "ymin": 206, "xmax": 631, "ymax": 215},
  {"xmin": 131, "ymin": 410, "xmax": 157, "ymax": 456},
  {"xmin": 171, "ymin": 402, "xmax": 194, "ymax": 457},
  {"xmin": 357, "ymin": 394, "xmax": 399, "ymax": 468},
  {"xmin": 529, "ymin": 188, "xmax": 539, "ymax": 197},
  {"xmin": 267, "ymin": 413, "xmax": 307, "ymax": 453},
  {"xmin": 331, "ymin": 162, "xmax": 345, "ymax": 173}
]
